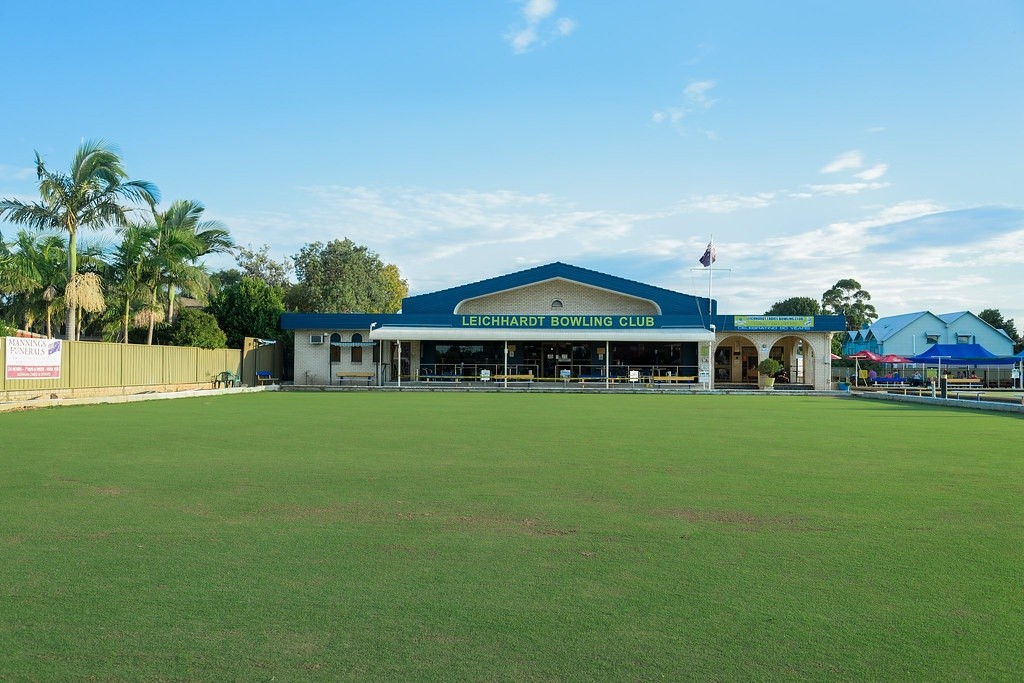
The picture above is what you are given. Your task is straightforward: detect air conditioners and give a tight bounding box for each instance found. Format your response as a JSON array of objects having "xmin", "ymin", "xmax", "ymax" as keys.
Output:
[{"xmin": 309, "ymin": 335, "xmax": 324, "ymax": 345}]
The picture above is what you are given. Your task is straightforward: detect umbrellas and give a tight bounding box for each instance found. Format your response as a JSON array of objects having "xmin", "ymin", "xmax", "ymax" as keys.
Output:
[
  {"xmin": 848, "ymin": 350, "xmax": 881, "ymax": 362},
  {"xmin": 830, "ymin": 353, "xmax": 843, "ymax": 360},
  {"xmin": 881, "ymin": 353, "xmax": 913, "ymax": 364}
]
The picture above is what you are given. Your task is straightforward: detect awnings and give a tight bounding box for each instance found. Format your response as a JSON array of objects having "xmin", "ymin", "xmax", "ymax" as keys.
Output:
[{"xmin": 907, "ymin": 343, "xmax": 1024, "ymax": 364}]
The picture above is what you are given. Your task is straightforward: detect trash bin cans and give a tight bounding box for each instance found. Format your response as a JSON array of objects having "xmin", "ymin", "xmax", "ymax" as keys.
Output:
[{"xmin": 838, "ymin": 382, "xmax": 849, "ymax": 393}]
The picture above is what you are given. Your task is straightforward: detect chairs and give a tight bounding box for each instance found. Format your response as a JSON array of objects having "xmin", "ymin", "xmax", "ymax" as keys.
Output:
[{"xmin": 215, "ymin": 371, "xmax": 241, "ymax": 388}]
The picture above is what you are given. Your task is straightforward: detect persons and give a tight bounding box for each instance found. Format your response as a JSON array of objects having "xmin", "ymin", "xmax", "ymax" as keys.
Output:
[{"xmin": 852, "ymin": 368, "xmax": 981, "ymax": 388}]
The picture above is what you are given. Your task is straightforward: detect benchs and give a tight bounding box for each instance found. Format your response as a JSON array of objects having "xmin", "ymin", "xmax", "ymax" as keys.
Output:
[
  {"xmin": 577, "ymin": 383, "xmax": 619, "ymax": 388},
  {"xmin": 941, "ymin": 377, "xmax": 985, "ymax": 390},
  {"xmin": 872, "ymin": 377, "xmax": 911, "ymax": 389},
  {"xmin": 335, "ymin": 370, "xmax": 375, "ymax": 388},
  {"xmin": 494, "ymin": 373, "xmax": 537, "ymax": 388},
  {"xmin": 256, "ymin": 371, "xmax": 283, "ymax": 386},
  {"xmin": 652, "ymin": 375, "xmax": 694, "ymax": 389},
  {"xmin": 421, "ymin": 381, "xmax": 462, "ymax": 391}
]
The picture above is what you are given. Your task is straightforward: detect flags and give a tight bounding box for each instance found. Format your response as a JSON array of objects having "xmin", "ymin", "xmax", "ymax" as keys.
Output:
[{"xmin": 699, "ymin": 237, "xmax": 717, "ymax": 267}]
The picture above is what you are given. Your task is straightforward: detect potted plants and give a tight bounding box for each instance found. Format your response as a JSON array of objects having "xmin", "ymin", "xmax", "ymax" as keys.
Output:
[
  {"xmin": 756, "ymin": 358, "xmax": 784, "ymax": 389},
  {"xmin": 844, "ymin": 382, "xmax": 852, "ymax": 393}
]
